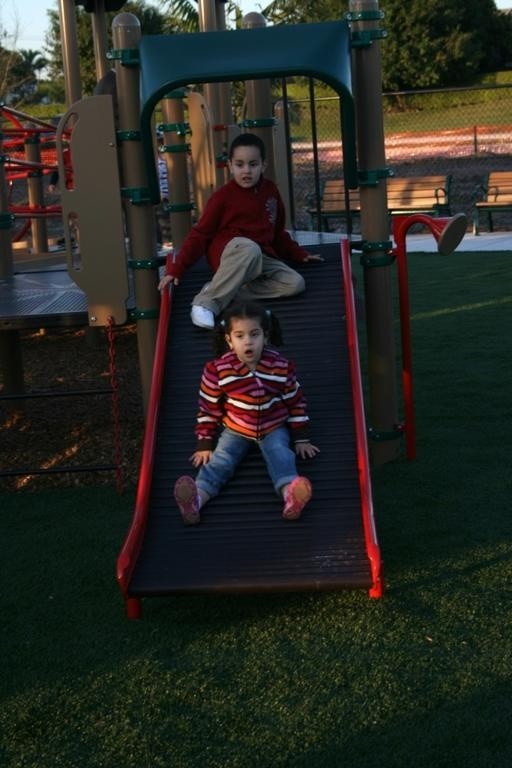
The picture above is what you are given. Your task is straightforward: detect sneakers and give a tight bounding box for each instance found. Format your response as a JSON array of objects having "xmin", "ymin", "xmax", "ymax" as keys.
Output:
[
  {"xmin": 191, "ymin": 305, "xmax": 214, "ymax": 328},
  {"xmin": 173, "ymin": 476, "xmax": 201, "ymax": 525},
  {"xmin": 284, "ymin": 477, "xmax": 311, "ymax": 520}
]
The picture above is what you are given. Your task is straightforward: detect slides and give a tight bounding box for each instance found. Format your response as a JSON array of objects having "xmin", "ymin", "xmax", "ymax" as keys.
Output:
[{"xmin": 115, "ymin": 237, "xmax": 384, "ymax": 619}]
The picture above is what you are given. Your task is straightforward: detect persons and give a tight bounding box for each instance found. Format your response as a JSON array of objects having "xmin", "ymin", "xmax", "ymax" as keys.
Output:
[
  {"xmin": 157, "ymin": 132, "xmax": 326, "ymax": 328},
  {"xmin": 172, "ymin": 302, "xmax": 321, "ymax": 526},
  {"xmin": 48, "ymin": 167, "xmax": 76, "ymax": 195},
  {"xmin": 156, "ymin": 132, "xmax": 172, "ymax": 246}
]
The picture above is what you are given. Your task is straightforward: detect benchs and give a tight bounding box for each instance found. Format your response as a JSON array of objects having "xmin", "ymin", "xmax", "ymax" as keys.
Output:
[
  {"xmin": 470, "ymin": 171, "xmax": 512, "ymax": 236},
  {"xmin": 304, "ymin": 171, "xmax": 455, "ymax": 232}
]
